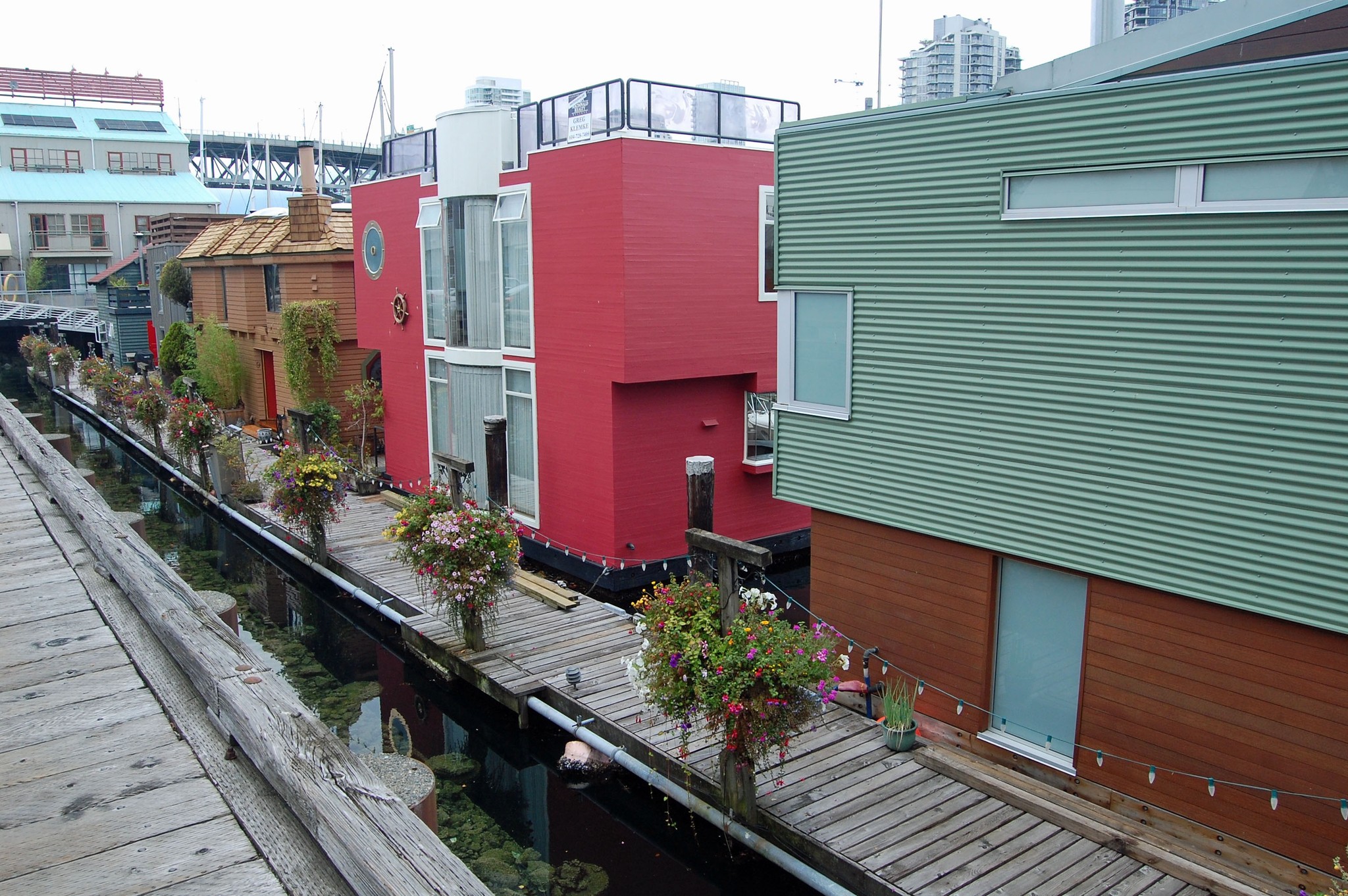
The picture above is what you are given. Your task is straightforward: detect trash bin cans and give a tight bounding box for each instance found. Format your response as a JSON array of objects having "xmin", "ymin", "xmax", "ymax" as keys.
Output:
[{"xmin": 123, "ymin": 361, "xmax": 138, "ymax": 376}]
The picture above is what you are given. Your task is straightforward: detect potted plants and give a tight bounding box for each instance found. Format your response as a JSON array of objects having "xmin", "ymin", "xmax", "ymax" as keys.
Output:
[
  {"xmin": 194, "ymin": 312, "xmax": 246, "ymax": 426},
  {"xmin": 277, "ymin": 300, "xmax": 343, "ymax": 446},
  {"xmin": 875, "ymin": 675, "xmax": 919, "ymax": 750}
]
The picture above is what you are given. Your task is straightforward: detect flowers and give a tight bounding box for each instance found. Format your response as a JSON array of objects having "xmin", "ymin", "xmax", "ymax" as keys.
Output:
[
  {"xmin": 19, "ymin": 334, "xmax": 522, "ymax": 639},
  {"xmin": 626, "ymin": 571, "xmax": 841, "ymax": 860},
  {"xmin": 138, "ymin": 279, "xmax": 149, "ymax": 287}
]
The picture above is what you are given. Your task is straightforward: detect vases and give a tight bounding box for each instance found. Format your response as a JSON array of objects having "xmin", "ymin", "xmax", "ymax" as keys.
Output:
[
  {"xmin": 137, "ymin": 285, "xmax": 151, "ymax": 290},
  {"xmin": 257, "ymin": 428, "xmax": 273, "ymax": 443},
  {"xmin": 338, "ymin": 470, "xmax": 379, "ymax": 495}
]
